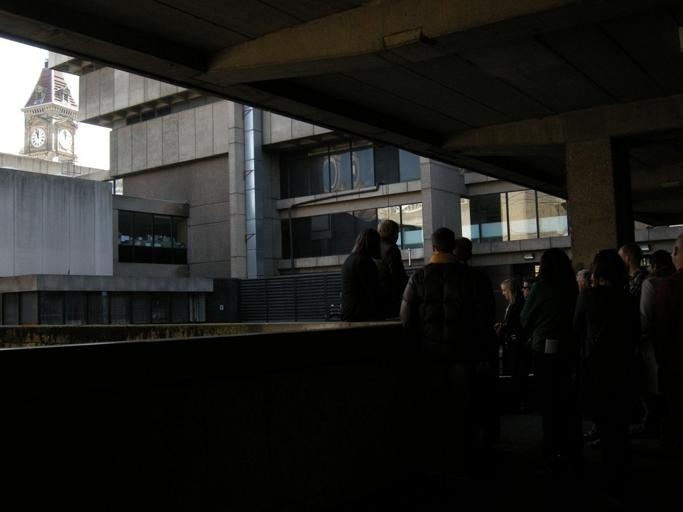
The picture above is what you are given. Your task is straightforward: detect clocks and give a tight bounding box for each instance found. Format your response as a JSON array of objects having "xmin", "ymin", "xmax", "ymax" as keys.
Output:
[{"xmin": 29, "ymin": 127, "xmax": 74, "ymax": 151}]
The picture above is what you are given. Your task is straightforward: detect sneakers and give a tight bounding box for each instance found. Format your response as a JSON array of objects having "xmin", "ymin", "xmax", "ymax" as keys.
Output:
[{"xmin": 582, "ymin": 422, "xmax": 675, "ymax": 450}]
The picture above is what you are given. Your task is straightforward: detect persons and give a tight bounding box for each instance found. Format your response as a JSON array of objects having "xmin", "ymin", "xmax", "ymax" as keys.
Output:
[
  {"xmin": 618, "ymin": 245, "xmax": 682, "ymax": 458},
  {"xmin": 400, "ymin": 226, "xmax": 493, "ymax": 510},
  {"xmin": 342, "ymin": 220, "xmax": 408, "ymax": 322},
  {"xmin": 519, "ymin": 248, "xmax": 641, "ymax": 508},
  {"xmin": 495, "ymin": 276, "xmax": 538, "ymax": 412}
]
